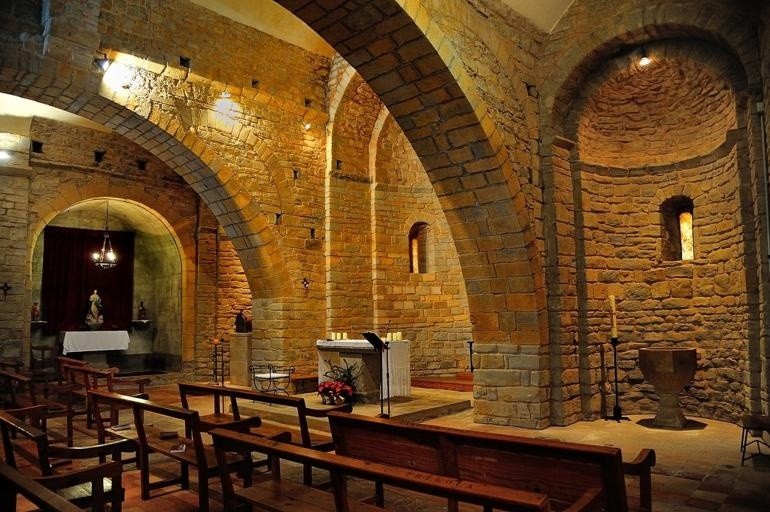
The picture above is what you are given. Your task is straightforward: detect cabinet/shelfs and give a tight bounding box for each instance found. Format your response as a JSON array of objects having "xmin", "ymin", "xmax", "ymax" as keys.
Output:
[{"xmin": 229, "ymin": 333, "xmax": 252, "ymax": 386}]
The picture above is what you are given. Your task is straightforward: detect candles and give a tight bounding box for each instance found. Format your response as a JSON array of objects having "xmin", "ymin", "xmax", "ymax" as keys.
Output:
[
  {"xmin": 332, "ymin": 332, "xmax": 347, "ymax": 340},
  {"xmin": 214, "ymin": 338, "xmax": 218, "ymax": 343},
  {"xmin": 608, "ymin": 295, "xmax": 617, "ymax": 338},
  {"xmin": 387, "ymin": 332, "xmax": 401, "ymax": 341}
]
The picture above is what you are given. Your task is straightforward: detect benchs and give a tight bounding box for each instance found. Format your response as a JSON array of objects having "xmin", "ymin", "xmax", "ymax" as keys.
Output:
[
  {"xmin": 175, "ymin": 381, "xmax": 352, "ymax": 487},
  {"xmin": 208, "ymin": 427, "xmax": 604, "ymax": 512},
  {"xmin": 87, "ymin": 390, "xmax": 261, "ymax": 501},
  {"xmin": 326, "ymin": 411, "xmax": 656, "ymax": 511},
  {"xmin": 0, "ymin": 358, "xmax": 151, "ymax": 512}
]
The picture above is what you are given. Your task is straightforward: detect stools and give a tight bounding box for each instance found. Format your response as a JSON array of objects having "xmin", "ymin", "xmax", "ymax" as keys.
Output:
[{"xmin": 736, "ymin": 414, "xmax": 770, "ymax": 466}]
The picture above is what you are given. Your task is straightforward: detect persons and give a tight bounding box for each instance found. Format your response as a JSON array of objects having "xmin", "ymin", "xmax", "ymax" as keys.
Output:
[{"xmin": 89, "ymin": 290, "xmax": 102, "ymax": 322}]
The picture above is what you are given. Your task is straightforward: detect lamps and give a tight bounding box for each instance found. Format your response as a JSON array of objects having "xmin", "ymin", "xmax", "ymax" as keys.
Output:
[
  {"xmin": 94, "ymin": 58, "xmax": 108, "ymax": 67},
  {"xmin": 639, "ymin": 48, "xmax": 650, "ymax": 66},
  {"xmin": 91, "ymin": 201, "xmax": 119, "ymax": 272}
]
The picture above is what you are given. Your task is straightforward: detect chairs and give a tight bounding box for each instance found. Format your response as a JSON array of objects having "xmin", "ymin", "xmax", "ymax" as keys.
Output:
[{"xmin": 30, "ymin": 343, "xmax": 62, "ymax": 386}]
